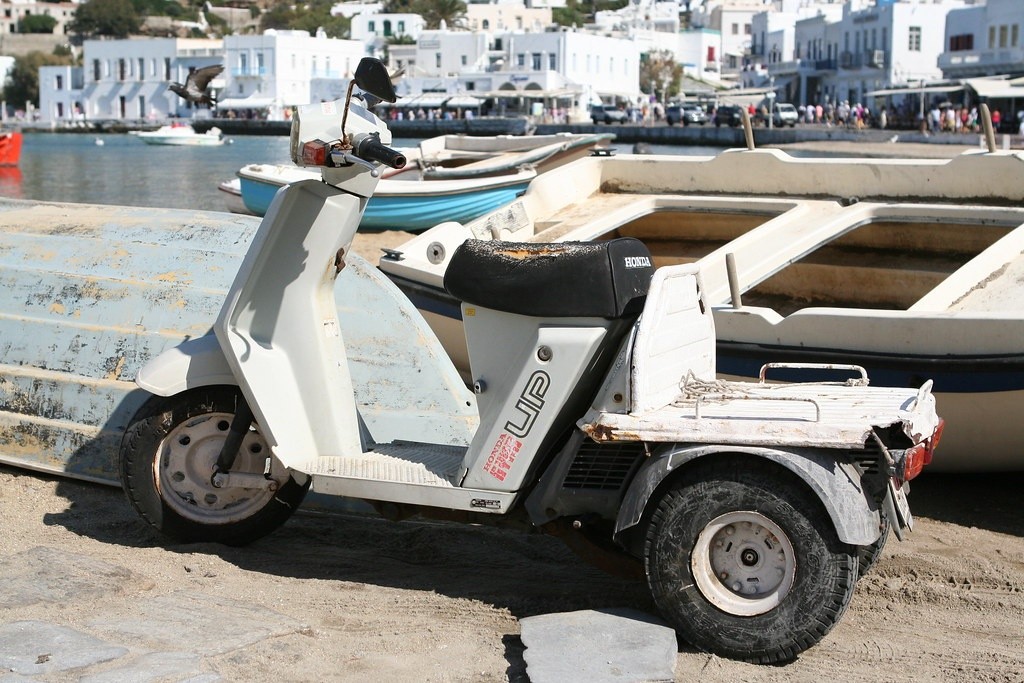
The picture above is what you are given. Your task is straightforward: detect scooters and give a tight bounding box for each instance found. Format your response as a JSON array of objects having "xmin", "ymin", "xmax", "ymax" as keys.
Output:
[{"xmin": 113, "ymin": 56, "xmax": 942, "ymax": 663}]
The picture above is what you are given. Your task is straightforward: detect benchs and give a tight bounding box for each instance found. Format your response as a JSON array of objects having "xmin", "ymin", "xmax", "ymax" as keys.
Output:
[{"xmin": 696, "ymin": 203, "xmax": 869, "ymax": 306}]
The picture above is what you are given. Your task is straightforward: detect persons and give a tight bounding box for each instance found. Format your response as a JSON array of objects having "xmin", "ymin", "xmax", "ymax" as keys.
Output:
[
  {"xmin": 919, "ymin": 103, "xmax": 1024, "ymax": 137},
  {"xmin": 799, "ymin": 101, "xmax": 889, "ymax": 130},
  {"xmin": 629, "ymin": 97, "xmax": 769, "ymax": 127},
  {"xmin": 541, "ymin": 106, "xmax": 573, "ymax": 126},
  {"xmin": 220, "ymin": 108, "xmax": 293, "ymax": 120},
  {"xmin": 379, "ymin": 108, "xmax": 472, "ymax": 120}
]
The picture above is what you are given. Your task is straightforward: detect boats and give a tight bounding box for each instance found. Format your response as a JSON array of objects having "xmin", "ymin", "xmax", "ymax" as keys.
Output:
[
  {"xmin": 219, "ymin": 161, "xmax": 421, "ymax": 216},
  {"xmin": 369, "ymin": 143, "xmax": 1024, "ymax": 483},
  {"xmin": 236, "ymin": 162, "xmax": 540, "ymax": 229},
  {"xmin": 127, "ymin": 122, "xmax": 226, "ymax": 146},
  {"xmin": 416, "ymin": 131, "xmax": 618, "ymax": 175},
  {"xmin": 0, "ymin": 129, "xmax": 25, "ymax": 169}
]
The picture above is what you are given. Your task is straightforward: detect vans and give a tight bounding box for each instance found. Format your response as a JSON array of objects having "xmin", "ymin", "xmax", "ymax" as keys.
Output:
[
  {"xmin": 714, "ymin": 106, "xmax": 743, "ymax": 127},
  {"xmin": 590, "ymin": 103, "xmax": 628, "ymax": 124},
  {"xmin": 666, "ymin": 105, "xmax": 709, "ymax": 127}
]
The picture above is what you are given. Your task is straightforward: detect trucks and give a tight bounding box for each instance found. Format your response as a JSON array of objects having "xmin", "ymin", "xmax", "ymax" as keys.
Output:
[{"xmin": 761, "ymin": 102, "xmax": 799, "ymax": 128}]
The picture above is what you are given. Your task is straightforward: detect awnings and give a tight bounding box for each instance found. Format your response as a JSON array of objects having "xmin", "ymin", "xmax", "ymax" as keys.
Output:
[
  {"xmin": 867, "ymin": 71, "xmax": 1024, "ymax": 100},
  {"xmin": 376, "ymin": 87, "xmax": 582, "ymax": 105}
]
